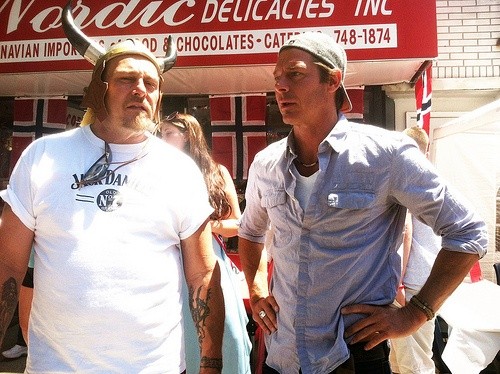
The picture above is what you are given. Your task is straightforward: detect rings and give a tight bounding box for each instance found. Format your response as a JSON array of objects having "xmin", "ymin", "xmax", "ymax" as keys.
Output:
[{"xmin": 259, "ymin": 311, "xmax": 266, "ymax": 319}]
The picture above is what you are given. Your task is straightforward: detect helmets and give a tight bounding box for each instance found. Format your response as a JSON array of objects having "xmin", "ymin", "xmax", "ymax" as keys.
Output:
[{"xmin": 62, "ymin": 1, "xmax": 178, "ymax": 121}]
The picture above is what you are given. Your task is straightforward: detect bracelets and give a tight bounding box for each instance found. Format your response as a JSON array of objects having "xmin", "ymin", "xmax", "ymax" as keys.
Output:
[{"xmin": 409, "ymin": 294, "xmax": 435, "ymax": 321}]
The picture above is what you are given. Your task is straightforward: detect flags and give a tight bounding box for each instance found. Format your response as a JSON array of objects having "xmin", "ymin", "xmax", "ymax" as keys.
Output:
[
  {"xmin": 342, "ymin": 89, "xmax": 363, "ymax": 123},
  {"xmin": 415, "ymin": 60, "xmax": 430, "ymax": 150},
  {"xmin": 12, "ymin": 100, "xmax": 67, "ymax": 178},
  {"xmin": 210, "ymin": 95, "xmax": 266, "ymax": 181}
]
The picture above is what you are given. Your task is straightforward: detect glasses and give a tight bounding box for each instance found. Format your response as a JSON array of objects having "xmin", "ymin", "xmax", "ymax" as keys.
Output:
[
  {"xmin": 163, "ymin": 111, "xmax": 179, "ymax": 121},
  {"xmin": 79, "ymin": 143, "xmax": 113, "ymax": 184}
]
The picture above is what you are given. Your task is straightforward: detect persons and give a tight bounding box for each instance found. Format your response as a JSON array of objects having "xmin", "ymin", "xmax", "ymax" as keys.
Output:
[
  {"xmin": 161, "ymin": 114, "xmax": 254, "ymax": 374},
  {"xmin": 234, "ymin": 34, "xmax": 487, "ymax": 374},
  {"xmin": 1, "ymin": 53, "xmax": 225, "ymax": 374},
  {"xmin": 0, "ymin": 244, "xmax": 35, "ymax": 360},
  {"xmin": 389, "ymin": 125, "xmax": 500, "ymax": 374}
]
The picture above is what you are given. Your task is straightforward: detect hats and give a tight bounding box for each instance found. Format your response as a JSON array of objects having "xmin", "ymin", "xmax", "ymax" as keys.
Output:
[{"xmin": 280, "ymin": 31, "xmax": 355, "ymax": 112}]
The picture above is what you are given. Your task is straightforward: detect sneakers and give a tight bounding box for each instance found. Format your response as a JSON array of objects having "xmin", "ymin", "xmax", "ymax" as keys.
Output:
[{"xmin": 1, "ymin": 344, "xmax": 28, "ymax": 358}]
